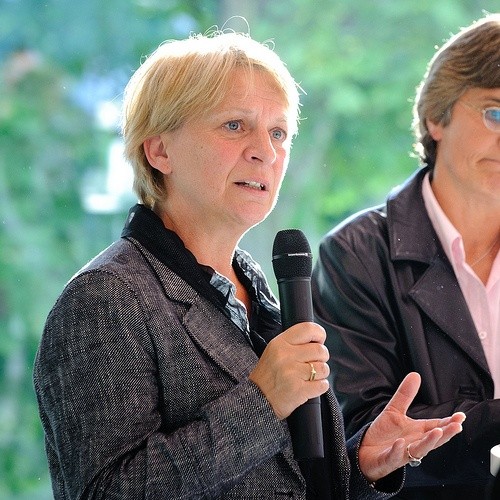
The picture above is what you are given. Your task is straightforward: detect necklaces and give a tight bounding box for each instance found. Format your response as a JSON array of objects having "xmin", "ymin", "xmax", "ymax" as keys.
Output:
[{"xmin": 470, "ymin": 238, "xmax": 498, "ymax": 268}]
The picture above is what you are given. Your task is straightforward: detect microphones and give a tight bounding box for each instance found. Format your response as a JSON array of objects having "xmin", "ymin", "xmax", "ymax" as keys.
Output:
[{"xmin": 271, "ymin": 228, "xmax": 326, "ymax": 464}]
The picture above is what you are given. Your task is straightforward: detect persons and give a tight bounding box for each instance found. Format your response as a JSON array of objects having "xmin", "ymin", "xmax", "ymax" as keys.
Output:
[
  {"xmin": 33, "ymin": 33, "xmax": 465, "ymax": 500},
  {"xmin": 310, "ymin": 12, "xmax": 500, "ymax": 500}
]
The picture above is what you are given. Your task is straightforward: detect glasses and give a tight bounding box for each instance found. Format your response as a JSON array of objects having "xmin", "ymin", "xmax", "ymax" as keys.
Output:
[{"xmin": 457, "ymin": 98, "xmax": 499, "ymax": 135}]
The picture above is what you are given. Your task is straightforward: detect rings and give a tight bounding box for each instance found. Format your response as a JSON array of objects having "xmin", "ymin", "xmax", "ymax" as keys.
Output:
[
  {"xmin": 309, "ymin": 361, "xmax": 316, "ymax": 382},
  {"xmin": 406, "ymin": 442, "xmax": 424, "ymax": 467}
]
